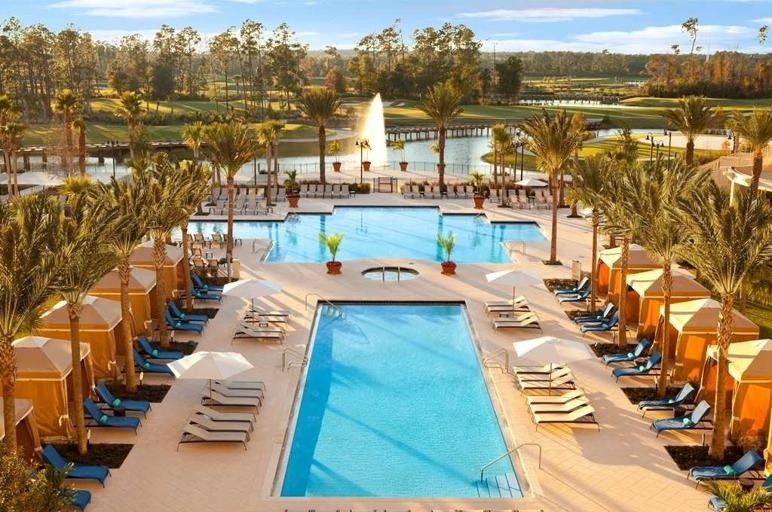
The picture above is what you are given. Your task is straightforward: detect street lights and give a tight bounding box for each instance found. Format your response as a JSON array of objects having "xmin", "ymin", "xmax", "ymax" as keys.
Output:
[
  {"xmin": 355, "ymin": 137, "xmax": 370, "ymax": 184},
  {"xmin": 645, "ymin": 125, "xmax": 672, "ymax": 173},
  {"xmin": 726, "ymin": 127, "xmax": 737, "ymax": 154},
  {"xmin": 103, "ymin": 136, "xmax": 121, "ymax": 180},
  {"xmin": 515, "ymin": 126, "xmax": 529, "ymax": 181}
]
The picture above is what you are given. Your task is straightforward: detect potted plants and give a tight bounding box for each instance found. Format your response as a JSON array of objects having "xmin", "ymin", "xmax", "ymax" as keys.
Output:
[
  {"xmin": 327, "ymin": 139, "xmax": 347, "ymax": 173},
  {"xmin": 282, "ymin": 169, "xmax": 302, "ymax": 208},
  {"xmin": 430, "ymin": 229, "xmax": 464, "ymax": 278},
  {"xmin": 389, "ymin": 138, "xmax": 409, "ymax": 172},
  {"xmin": 356, "ymin": 138, "xmax": 372, "ymax": 171},
  {"xmin": 470, "ymin": 168, "xmax": 491, "ymax": 209},
  {"xmin": 317, "ymin": 229, "xmax": 350, "ymax": 278},
  {"xmin": 430, "ymin": 142, "xmax": 446, "ymax": 174}
]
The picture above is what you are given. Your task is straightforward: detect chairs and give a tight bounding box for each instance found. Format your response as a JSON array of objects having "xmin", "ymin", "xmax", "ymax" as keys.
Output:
[
  {"xmin": 402, "ymin": 184, "xmax": 475, "ymax": 200},
  {"xmin": 204, "ymin": 181, "xmax": 350, "ymax": 216},
  {"xmin": 162, "ymin": 230, "xmax": 228, "ymax": 267},
  {"xmin": 488, "ymin": 182, "xmax": 553, "ymax": 210},
  {"xmin": 513, "ymin": 360, "xmax": 600, "ymax": 431},
  {"xmin": 483, "ymin": 295, "xmax": 542, "ymax": 331},
  {"xmin": 175, "ymin": 376, "xmax": 267, "ymax": 451},
  {"xmin": 232, "ymin": 302, "xmax": 289, "ymax": 345}
]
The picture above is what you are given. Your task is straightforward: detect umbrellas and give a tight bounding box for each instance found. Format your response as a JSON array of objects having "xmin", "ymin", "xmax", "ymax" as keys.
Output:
[
  {"xmin": 222, "ymin": 274, "xmax": 283, "ymax": 325},
  {"xmin": 485, "ymin": 266, "xmax": 543, "ymax": 313},
  {"xmin": 511, "ymin": 333, "xmax": 593, "ymax": 395},
  {"xmin": 166, "ymin": 351, "xmax": 256, "ymax": 410}
]
